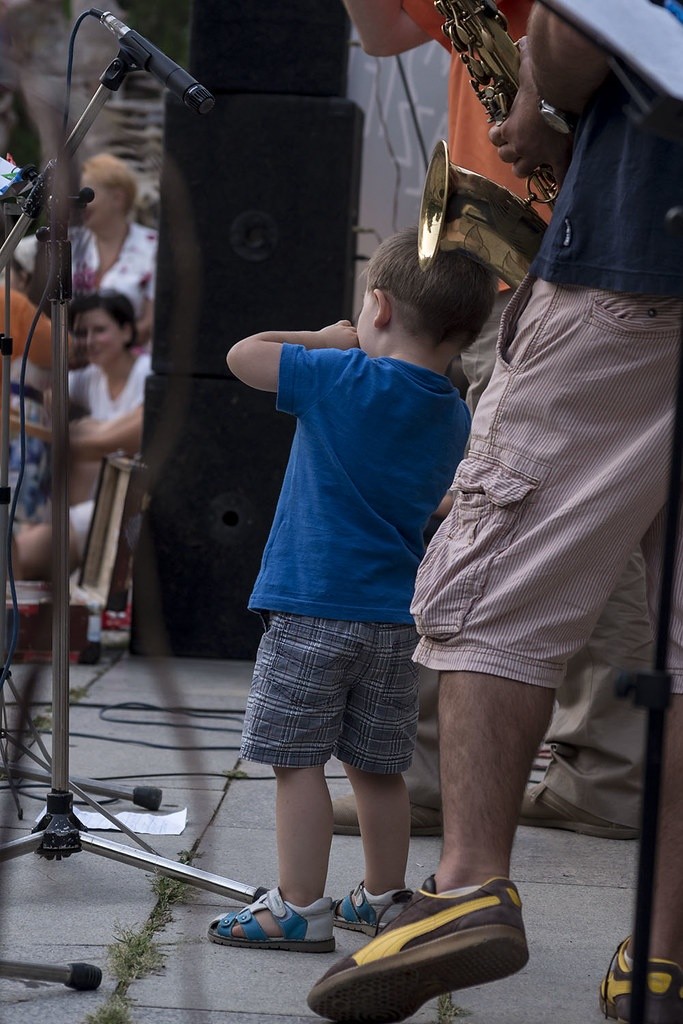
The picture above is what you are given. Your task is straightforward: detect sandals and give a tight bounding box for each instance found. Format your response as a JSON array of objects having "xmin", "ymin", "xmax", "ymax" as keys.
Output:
[
  {"xmin": 206, "ymin": 884, "xmax": 335, "ymax": 953},
  {"xmin": 332, "ymin": 880, "xmax": 414, "ymax": 938}
]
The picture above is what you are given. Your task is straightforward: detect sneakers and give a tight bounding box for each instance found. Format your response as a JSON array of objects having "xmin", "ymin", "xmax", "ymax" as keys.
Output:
[
  {"xmin": 305, "ymin": 875, "xmax": 529, "ymax": 1024},
  {"xmin": 516, "ymin": 783, "xmax": 640, "ymax": 841},
  {"xmin": 331, "ymin": 793, "xmax": 444, "ymax": 837},
  {"xmin": 599, "ymin": 935, "xmax": 683, "ymax": 1024}
]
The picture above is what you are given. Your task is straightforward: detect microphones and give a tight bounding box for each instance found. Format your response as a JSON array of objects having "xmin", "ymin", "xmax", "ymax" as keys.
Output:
[{"xmin": 100, "ymin": 11, "xmax": 215, "ymax": 115}]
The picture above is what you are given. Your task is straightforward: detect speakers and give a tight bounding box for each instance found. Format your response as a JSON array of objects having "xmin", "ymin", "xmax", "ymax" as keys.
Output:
[{"xmin": 130, "ymin": 0, "xmax": 367, "ymax": 658}]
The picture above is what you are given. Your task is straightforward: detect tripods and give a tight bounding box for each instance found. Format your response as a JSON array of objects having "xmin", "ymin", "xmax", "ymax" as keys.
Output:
[{"xmin": 0, "ymin": 48, "xmax": 271, "ymax": 905}]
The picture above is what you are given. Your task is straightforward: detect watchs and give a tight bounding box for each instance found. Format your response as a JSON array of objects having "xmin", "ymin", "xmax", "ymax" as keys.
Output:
[{"xmin": 538, "ymin": 99, "xmax": 571, "ymax": 135}]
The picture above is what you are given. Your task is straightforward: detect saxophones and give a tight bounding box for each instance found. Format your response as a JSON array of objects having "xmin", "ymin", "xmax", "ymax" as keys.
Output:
[{"xmin": 420, "ymin": 0, "xmax": 573, "ymax": 285}]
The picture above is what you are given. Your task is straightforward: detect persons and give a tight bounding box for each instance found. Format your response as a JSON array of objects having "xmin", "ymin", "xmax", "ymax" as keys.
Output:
[
  {"xmin": 207, "ymin": 219, "xmax": 503, "ymax": 954},
  {"xmin": 0, "ymin": 153, "xmax": 161, "ymax": 669},
  {"xmin": 304, "ymin": 0, "xmax": 683, "ymax": 1024}
]
